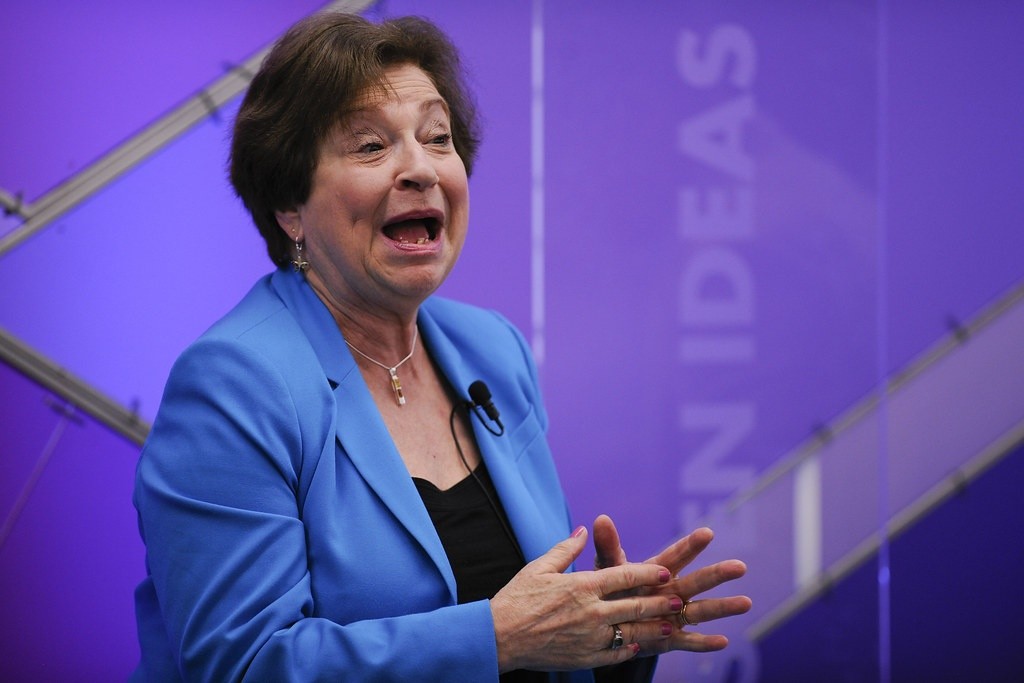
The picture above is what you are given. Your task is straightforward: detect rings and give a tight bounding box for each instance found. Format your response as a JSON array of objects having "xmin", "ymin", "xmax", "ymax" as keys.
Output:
[
  {"xmin": 681, "ymin": 601, "xmax": 698, "ymax": 626},
  {"xmin": 610, "ymin": 625, "xmax": 624, "ymax": 650}
]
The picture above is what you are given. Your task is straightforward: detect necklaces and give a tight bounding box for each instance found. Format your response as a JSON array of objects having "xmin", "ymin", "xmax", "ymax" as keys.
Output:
[{"xmin": 345, "ymin": 325, "xmax": 418, "ymax": 405}]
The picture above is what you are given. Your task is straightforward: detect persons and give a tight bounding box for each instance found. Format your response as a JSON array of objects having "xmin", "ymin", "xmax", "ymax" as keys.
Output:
[{"xmin": 126, "ymin": 12, "xmax": 752, "ymax": 683}]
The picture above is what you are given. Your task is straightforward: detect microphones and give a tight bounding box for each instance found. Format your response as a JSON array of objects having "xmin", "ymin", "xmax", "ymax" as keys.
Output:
[{"xmin": 469, "ymin": 379, "xmax": 500, "ymax": 421}]
